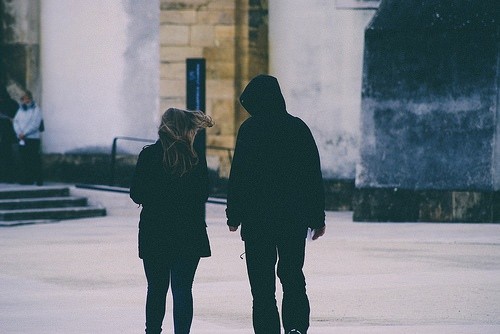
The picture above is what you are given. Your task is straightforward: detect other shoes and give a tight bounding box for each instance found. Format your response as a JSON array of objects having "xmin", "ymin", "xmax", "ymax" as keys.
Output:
[
  {"xmin": 37, "ymin": 178, "xmax": 43, "ymax": 185},
  {"xmin": 19, "ymin": 178, "xmax": 33, "ymax": 184}
]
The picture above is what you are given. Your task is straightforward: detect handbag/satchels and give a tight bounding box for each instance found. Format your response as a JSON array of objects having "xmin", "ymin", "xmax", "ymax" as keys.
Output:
[{"xmin": 39, "ymin": 119, "xmax": 44, "ymax": 132}]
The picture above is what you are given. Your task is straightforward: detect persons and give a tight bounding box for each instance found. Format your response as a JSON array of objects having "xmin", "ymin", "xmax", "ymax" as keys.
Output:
[
  {"xmin": 12, "ymin": 90, "xmax": 45, "ymax": 188},
  {"xmin": 225, "ymin": 74, "xmax": 327, "ymax": 334},
  {"xmin": 129, "ymin": 107, "xmax": 216, "ymax": 334}
]
